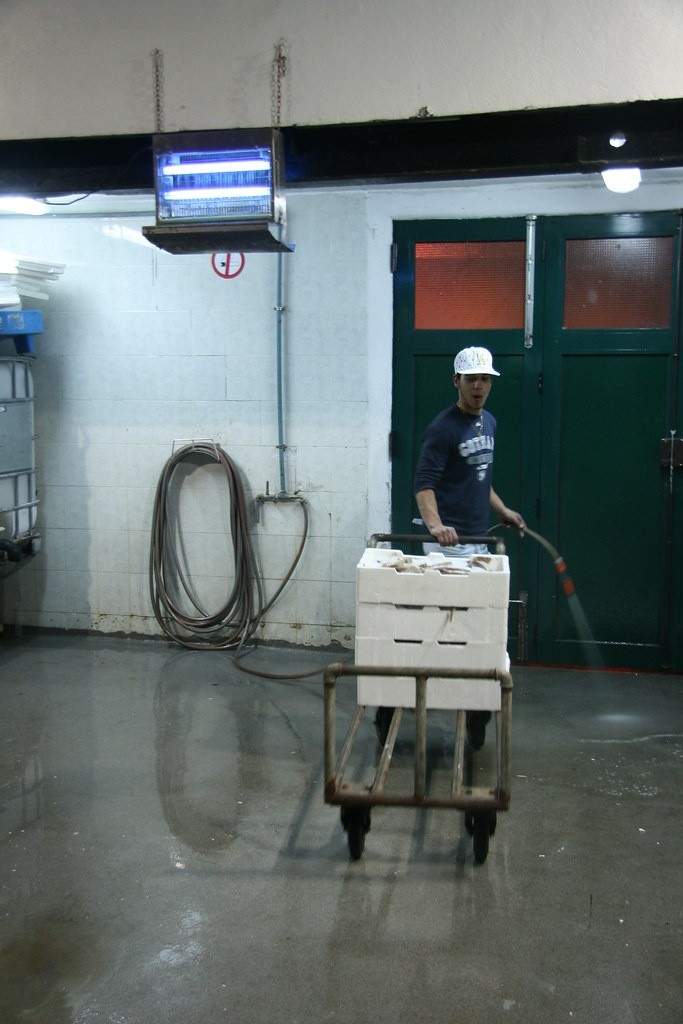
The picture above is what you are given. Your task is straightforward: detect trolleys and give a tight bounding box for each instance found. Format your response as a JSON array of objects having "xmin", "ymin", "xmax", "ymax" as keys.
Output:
[{"xmin": 323, "ymin": 533, "xmax": 523, "ymax": 863}]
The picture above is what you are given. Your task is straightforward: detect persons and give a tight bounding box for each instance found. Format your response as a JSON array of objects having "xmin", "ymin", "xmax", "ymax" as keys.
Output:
[{"xmin": 415, "ymin": 346, "xmax": 526, "ymax": 559}]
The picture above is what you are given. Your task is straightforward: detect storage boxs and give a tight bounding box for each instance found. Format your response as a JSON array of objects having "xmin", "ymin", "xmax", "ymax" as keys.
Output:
[{"xmin": 355, "ymin": 548, "xmax": 511, "ymax": 710}]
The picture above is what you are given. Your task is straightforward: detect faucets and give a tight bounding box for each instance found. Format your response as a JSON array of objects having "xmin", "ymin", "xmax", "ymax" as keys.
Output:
[{"xmin": 290, "ymin": 489, "xmax": 303, "ymax": 500}]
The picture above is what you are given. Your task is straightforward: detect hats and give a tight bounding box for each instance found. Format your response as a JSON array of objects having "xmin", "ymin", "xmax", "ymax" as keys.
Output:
[{"xmin": 454, "ymin": 346, "xmax": 501, "ymax": 376}]
